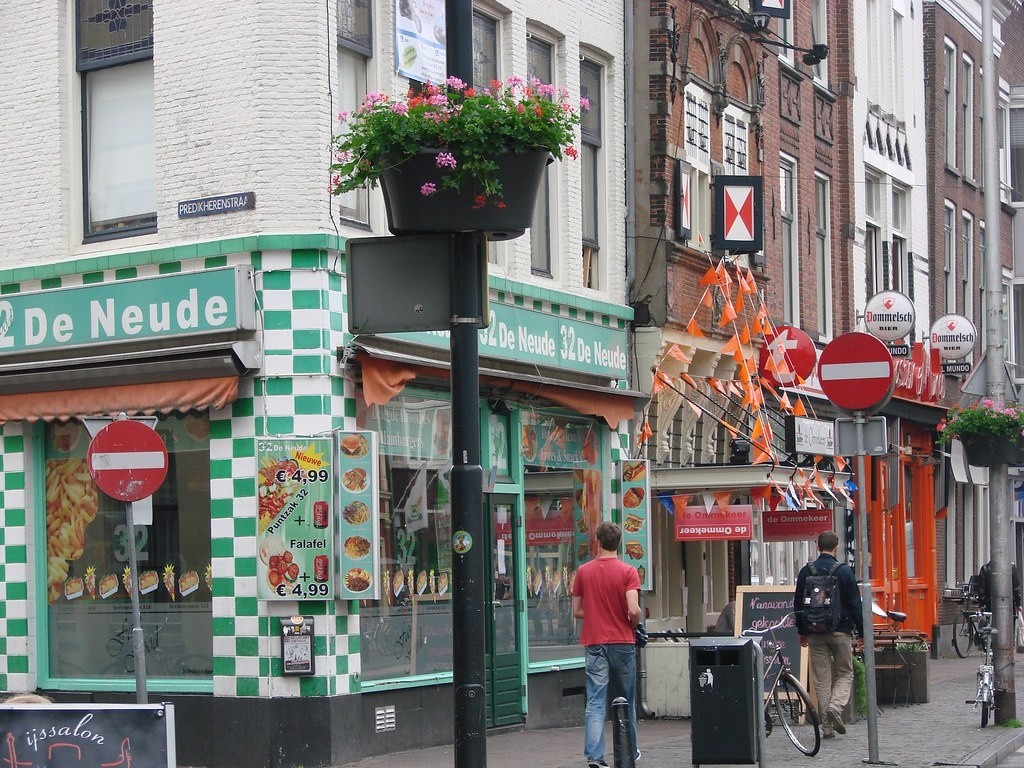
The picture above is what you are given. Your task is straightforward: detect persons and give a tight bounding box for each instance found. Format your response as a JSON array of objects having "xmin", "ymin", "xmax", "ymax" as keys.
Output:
[
  {"xmin": 570, "ymin": 521, "xmax": 642, "ymax": 768},
  {"xmin": 978, "ymin": 560, "xmax": 1023, "ymax": 663},
  {"xmin": 794, "ymin": 531, "xmax": 864, "ymax": 739},
  {"xmin": 714, "ymin": 602, "xmax": 736, "ymax": 634}
]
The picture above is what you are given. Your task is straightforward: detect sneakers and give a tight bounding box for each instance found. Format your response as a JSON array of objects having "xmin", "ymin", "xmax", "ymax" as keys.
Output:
[
  {"xmin": 823, "ymin": 731, "xmax": 836, "ymax": 739},
  {"xmin": 826, "ymin": 707, "xmax": 846, "ymax": 734}
]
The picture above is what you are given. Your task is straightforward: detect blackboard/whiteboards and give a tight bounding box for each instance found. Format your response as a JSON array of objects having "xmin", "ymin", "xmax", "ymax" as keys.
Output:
[
  {"xmin": 734, "ymin": 585, "xmax": 816, "ymax": 700},
  {"xmin": 0, "ymin": 703, "xmax": 177, "ymax": 768}
]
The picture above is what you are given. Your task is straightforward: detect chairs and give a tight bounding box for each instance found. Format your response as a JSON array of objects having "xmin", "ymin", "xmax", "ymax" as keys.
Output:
[{"xmin": 874, "ymin": 635, "xmax": 913, "ymax": 709}]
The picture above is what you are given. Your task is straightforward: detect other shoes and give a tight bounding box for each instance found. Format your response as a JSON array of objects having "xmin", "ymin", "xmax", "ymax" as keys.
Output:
[
  {"xmin": 588, "ymin": 759, "xmax": 609, "ymax": 768},
  {"xmin": 634, "ymin": 750, "xmax": 642, "ymax": 762}
]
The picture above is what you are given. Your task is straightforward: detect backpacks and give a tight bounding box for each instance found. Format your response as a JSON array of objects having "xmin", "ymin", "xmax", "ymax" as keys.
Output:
[{"xmin": 802, "ymin": 561, "xmax": 846, "ymax": 634}]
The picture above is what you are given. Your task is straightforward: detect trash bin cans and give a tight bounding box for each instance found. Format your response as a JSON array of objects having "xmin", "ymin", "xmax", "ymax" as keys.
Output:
[{"xmin": 688, "ymin": 637, "xmax": 759, "ymax": 764}]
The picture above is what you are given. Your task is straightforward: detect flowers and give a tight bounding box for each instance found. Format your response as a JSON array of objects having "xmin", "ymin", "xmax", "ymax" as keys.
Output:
[
  {"xmin": 319, "ymin": 76, "xmax": 591, "ymax": 212},
  {"xmin": 933, "ymin": 392, "xmax": 1024, "ymax": 448}
]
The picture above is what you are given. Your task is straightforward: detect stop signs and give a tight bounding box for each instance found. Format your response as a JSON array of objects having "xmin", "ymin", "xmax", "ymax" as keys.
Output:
[
  {"xmin": 88, "ymin": 420, "xmax": 168, "ymax": 502},
  {"xmin": 817, "ymin": 333, "xmax": 894, "ymax": 408}
]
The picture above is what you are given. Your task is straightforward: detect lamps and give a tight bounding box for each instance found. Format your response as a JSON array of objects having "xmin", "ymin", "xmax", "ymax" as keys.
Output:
[
  {"xmin": 750, "ymin": 9, "xmax": 771, "ymax": 36},
  {"xmin": 751, "ymin": 34, "xmax": 829, "ymax": 66}
]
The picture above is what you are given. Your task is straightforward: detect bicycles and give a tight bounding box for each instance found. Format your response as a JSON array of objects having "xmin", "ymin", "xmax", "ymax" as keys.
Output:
[
  {"xmin": 736, "ymin": 609, "xmax": 822, "ymax": 757},
  {"xmin": 873, "ymin": 610, "xmax": 929, "ymax": 654},
  {"xmin": 965, "ymin": 612, "xmax": 995, "ymax": 728},
  {"xmin": 953, "ymin": 582, "xmax": 989, "ymax": 658}
]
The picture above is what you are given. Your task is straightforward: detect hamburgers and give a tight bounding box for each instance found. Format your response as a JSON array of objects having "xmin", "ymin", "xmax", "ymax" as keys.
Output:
[{"xmin": 340, "ymin": 435, "xmax": 363, "ymax": 456}]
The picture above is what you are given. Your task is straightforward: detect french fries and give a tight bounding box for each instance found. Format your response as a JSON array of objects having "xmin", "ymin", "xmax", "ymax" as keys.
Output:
[{"xmin": 46, "ymin": 458, "xmax": 99, "ymax": 603}]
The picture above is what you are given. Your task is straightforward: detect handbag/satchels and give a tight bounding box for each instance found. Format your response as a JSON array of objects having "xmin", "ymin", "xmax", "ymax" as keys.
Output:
[{"xmin": 1017, "ymin": 611, "xmax": 1024, "ymax": 653}]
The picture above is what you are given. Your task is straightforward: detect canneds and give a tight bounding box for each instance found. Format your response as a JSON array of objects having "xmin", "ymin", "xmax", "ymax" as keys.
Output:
[
  {"xmin": 313, "ymin": 500, "xmax": 330, "ymax": 530},
  {"xmin": 313, "ymin": 554, "xmax": 330, "ymax": 584}
]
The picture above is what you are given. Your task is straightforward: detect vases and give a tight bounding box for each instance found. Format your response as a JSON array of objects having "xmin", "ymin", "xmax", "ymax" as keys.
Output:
[
  {"xmin": 372, "ymin": 140, "xmax": 554, "ymax": 241},
  {"xmin": 962, "ymin": 433, "xmax": 1016, "ymax": 467}
]
